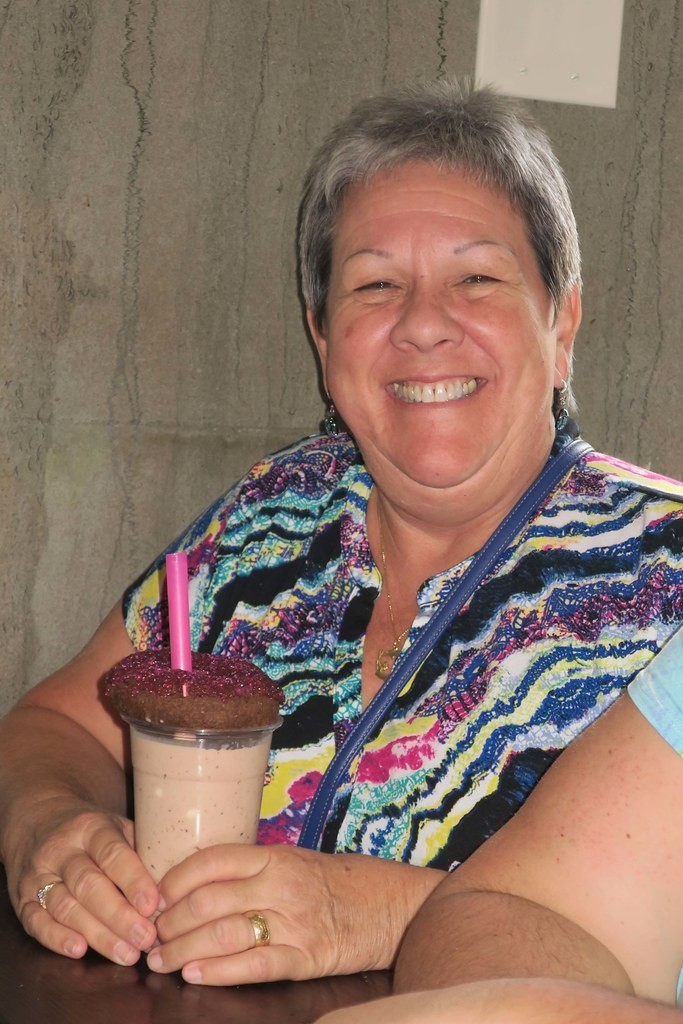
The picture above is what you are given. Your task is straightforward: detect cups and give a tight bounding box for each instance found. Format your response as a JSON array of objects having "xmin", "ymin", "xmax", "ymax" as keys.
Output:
[{"xmin": 120, "ymin": 713, "xmax": 283, "ymax": 955}]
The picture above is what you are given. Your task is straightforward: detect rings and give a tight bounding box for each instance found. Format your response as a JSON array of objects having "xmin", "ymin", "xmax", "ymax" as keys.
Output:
[
  {"xmin": 37, "ymin": 881, "xmax": 63, "ymax": 910},
  {"xmin": 244, "ymin": 911, "xmax": 269, "ymax": 948}
]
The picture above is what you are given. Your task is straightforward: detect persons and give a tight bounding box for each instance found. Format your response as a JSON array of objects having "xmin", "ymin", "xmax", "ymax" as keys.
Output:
[
  {"xmin": 0, "ymin": 90, "xmax": 683, "ymax": 986},
  {"xmin": 314, "ymin": 620, "xmax": 683, "ymax": 1024}
]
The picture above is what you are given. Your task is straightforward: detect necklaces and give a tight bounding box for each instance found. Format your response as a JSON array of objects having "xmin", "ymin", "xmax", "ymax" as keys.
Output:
[{"xmin": 375, "ymin": 501, "xmax": 410, "ymax": 680}]
